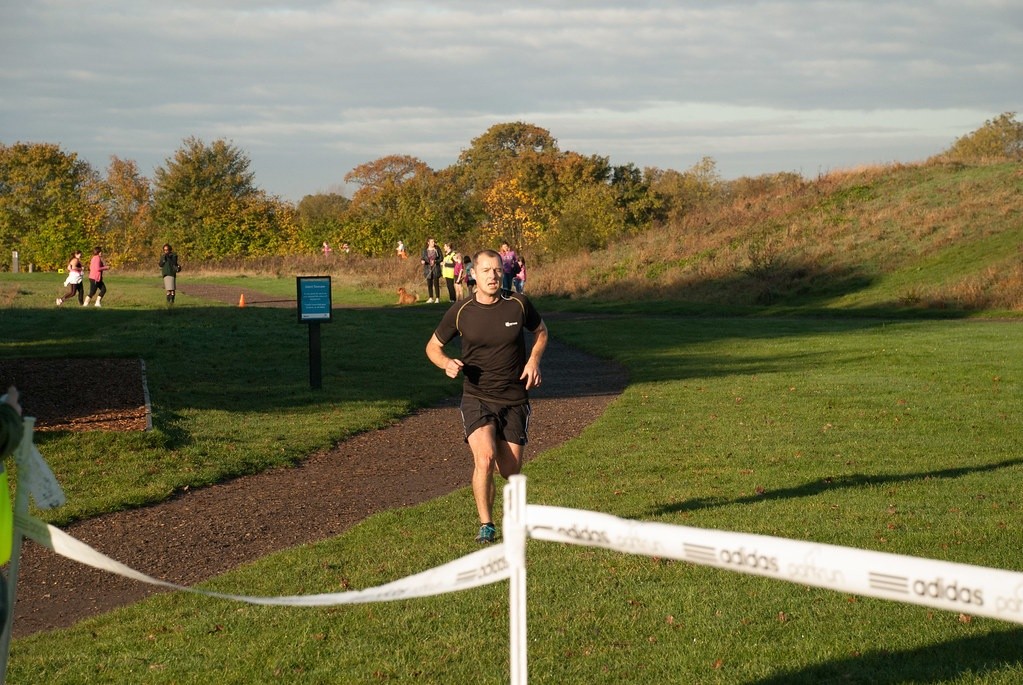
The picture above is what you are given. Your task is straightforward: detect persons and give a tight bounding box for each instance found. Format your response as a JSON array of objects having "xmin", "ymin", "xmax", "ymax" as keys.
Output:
[
  {"xmin": 462, "ymin": 255, "xmax": 475, "ymax": 299},
  {"xmin": 452, "ymin": 252, "xmax": 465, "ymax": 303},
  {"xmin": 420, "ymin": 237, "xmax": 443, "ymax": 304},
  {"xmin": 395, "ymin": 240, "xmax": 406, "ymax": 261},
  {"xmin": 440, "ymin": 243, "xmax": 457, "ymax": 304},
  {"xmin": 423, "ymin": 248, "xmax": 549, "ymax": 542},
  {"xmin": 55, "ymin": 249, "xmax": 86, "ymax": 307},
  {"xmin": 321, "ymin": 240, "xmax": 351, "ymax": 255},
  {"xmin": 78, "ymin": 246, "xmax": 110, "ymax": 307},
  {"xmin": 0, "ymin": 384, "xmax": 26, "ymax": 639},
  {"xmin": 497, "ymin": 241, "xmax": 516, "ymax": 297},
  {"xmin": 512, "ymin": 255, "xmax": 527, "ymax": 297},
  {"xmin": 158, "ymin": 243, "xmax": 182, "ymax": 304}
]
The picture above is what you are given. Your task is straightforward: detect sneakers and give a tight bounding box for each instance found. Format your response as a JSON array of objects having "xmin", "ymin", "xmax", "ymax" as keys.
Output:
[{"xmin": 475, "ymin": 527, "xmax": 495, "ymax": 543}]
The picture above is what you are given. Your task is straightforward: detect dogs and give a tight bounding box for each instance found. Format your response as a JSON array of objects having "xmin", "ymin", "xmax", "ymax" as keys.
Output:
[{"xmin": 392, "ymin": 287, "xmax": 420, "ymax": 305}]
